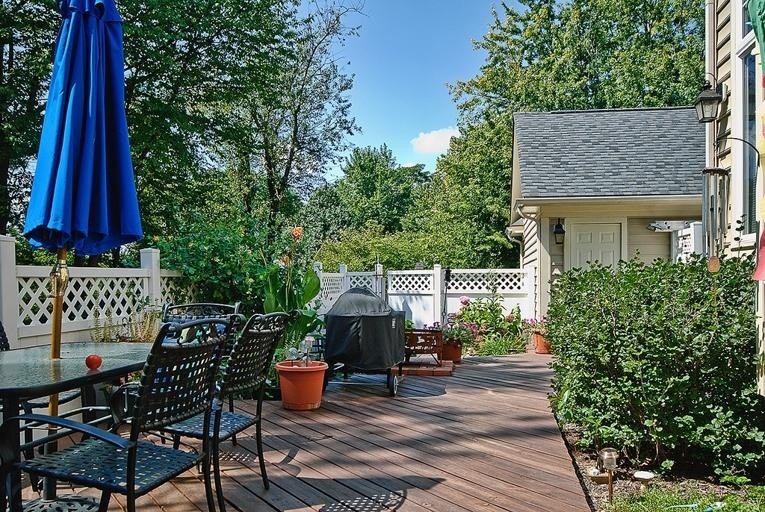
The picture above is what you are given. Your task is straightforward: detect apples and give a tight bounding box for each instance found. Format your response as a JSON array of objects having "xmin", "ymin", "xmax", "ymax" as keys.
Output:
[{"xmin": 85, "ymin": 355, "xmax": 102, "ymax": 369}]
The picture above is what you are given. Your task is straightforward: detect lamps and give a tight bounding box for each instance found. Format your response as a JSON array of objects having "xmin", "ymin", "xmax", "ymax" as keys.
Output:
[
  {"xmin": 553, "ymin": 218, "xmax": 565, "ymax": 244},
  {"xmin": 703, "ymin": 139, "xmax": 760, "ymax": 175},
  {"xmin": 694, "ymin": 73, "xmax": 727, "ymax": 123}
]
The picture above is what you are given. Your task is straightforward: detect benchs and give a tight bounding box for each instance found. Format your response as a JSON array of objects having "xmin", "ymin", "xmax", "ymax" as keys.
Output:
[{"xmin": 404, "ymin": 329, "xmax": 442, "ymax": 367}]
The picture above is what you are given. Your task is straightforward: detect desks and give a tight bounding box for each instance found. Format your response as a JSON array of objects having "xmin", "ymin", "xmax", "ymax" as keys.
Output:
[{"xmin": 1, "ymin": 338, "xmax": 225, "ymax": 512}]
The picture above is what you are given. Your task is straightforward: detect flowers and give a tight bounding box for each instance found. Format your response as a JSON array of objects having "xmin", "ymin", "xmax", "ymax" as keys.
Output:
[
  {"xmin": 286, "ymin": 336, "xmax": 315, "ymax": 367},
  {"xmin": 522, "ymin": 318, "xmax": 544, "ymax": 335},
  {"xmin": 436, "ymin": 295, "xmax": 479, "ymax": 345}
]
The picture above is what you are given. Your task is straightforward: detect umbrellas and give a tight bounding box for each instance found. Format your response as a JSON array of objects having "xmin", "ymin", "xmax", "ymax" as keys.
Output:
[{"xmin": 21, "ymin": 1, "xmax": 145, "ymax": 502}]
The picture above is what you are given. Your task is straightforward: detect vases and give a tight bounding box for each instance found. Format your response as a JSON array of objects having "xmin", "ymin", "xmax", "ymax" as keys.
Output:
[
  {"xmin": 438, "ymin": 340, "xmax": 461, "ymax": 362},
  {"xmin": 533, "ymin": 333, "xmax": 549, "ymax": 354},
  {"xmin": 275, "ymin": 361, "xmax": 329, "ymax": 411}
]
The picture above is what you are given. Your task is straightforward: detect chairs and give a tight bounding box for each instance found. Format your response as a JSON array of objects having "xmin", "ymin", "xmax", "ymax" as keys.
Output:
[
  {"xmin": 152, "ymin": 311, "xmax": 294, "ymax": 508},
  {"xmin": 116, "ymin": 301, "xmax": 244, "ymax": 448},
  {"xmin": 1, "ymin": 321, "xmax": 90, "ymax": 498}
]
